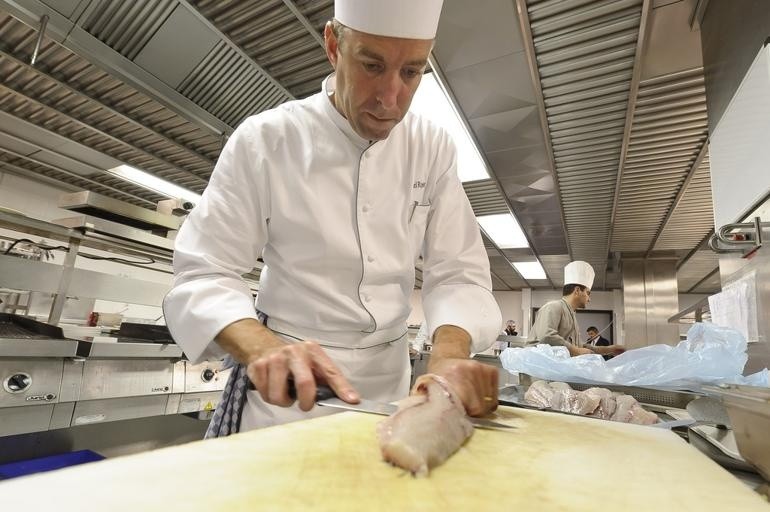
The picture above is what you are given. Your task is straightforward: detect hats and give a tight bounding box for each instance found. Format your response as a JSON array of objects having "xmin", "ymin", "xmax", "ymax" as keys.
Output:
[
  {"xmin": 334, "ymin": 0, "xmax": 444, "ymax": 41},
  {"xmin": 564, "ymin": 260, "xmax": 595, "ymax": 290}
]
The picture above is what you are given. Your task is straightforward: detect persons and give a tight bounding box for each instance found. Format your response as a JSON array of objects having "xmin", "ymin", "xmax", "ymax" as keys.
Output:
[
  {"xmin": 494, "ymin": 320, "xmax": 519, "ymax": 355},
  {"xmin": 161, "ymin": 0, "xmax": 505, "ymax": 441},
  {"xmin": 520, "ymin": 283, "xmax": 626, "ymax": 388},
  {"xmin": 586, "ymin": 326, "xmax": 611, "ymax": 360},
  {"xmin": 410, "ymin": 318, "xmax": 430, "ymax": 358}
]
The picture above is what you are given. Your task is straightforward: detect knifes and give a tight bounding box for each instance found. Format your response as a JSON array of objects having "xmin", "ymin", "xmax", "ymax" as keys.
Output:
[{"xmin": 241, "ymin": 373, "xmax": 519, "ymax": 433}]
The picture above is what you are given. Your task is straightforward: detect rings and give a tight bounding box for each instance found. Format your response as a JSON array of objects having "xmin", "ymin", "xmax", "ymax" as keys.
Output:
[{"xmin": 484, "ymin": 394, "xmax": 494, "ymax": 402}]
